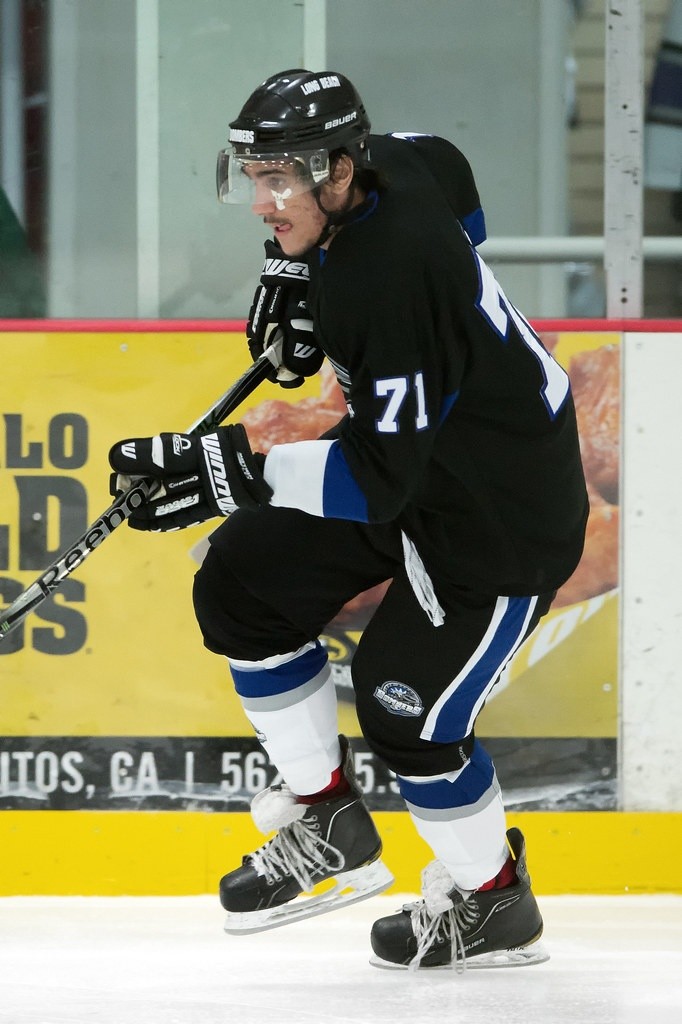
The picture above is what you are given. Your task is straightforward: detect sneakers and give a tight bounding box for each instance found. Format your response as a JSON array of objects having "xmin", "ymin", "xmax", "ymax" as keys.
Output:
[
  {"xmin": 368, "ymin": 828, "xmax": 549, "ymax": 973},
  {"xmin": 219, "ymin": 734, "xmax": 394, "ymax": 929}
]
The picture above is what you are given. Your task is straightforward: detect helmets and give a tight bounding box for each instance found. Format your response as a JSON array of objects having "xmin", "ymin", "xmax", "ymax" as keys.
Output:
[{"xmin": 216, "ymin": 69, "xmax": 371, "ymax": 206}]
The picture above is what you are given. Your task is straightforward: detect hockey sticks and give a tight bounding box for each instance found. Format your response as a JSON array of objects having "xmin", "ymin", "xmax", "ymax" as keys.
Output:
[{"xmin": 1, "ymin": 335, "xmax": 284, "ymax": 638}]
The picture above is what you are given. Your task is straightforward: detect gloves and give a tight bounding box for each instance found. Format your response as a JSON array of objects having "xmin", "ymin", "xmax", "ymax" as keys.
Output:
[
  {"xmin": 109, "ymin": 423, "xmax": 274, "ymax": 532},
  {"xmin": 246, "ymin": 240, "xmax": 326, "ymax": 389}
]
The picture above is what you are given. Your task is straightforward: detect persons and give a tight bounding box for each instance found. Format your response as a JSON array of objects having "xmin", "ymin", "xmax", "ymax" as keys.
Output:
[{"xmin": 110, "ymin": 69, "xmax": 589, "ymax": 969}]
